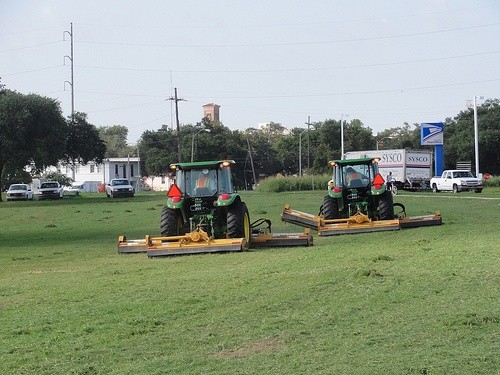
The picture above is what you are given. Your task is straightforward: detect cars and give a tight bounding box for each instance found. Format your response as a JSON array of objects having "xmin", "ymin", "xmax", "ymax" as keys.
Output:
[
  {"xmin": 5, "ymin": 184, "xmax": 34, "ymax": 201},
  {"xmin": 37, "ymin": 182, "xmax": 64, "ymax": 200}
]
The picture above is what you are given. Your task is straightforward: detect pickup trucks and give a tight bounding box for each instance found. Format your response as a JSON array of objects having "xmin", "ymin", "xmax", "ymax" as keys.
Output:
[
  {"xmin": 429, "ymin": 169, "xmax": 484, "ymax": 194},
  {"xmin": 105, "ymin": 178, "xmax": 135, "ymax": 199}
]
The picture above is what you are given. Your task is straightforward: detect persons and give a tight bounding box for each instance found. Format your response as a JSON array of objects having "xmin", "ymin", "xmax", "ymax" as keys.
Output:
[
  {"xmin": 387, "ymin": 172, "xmax": 395, "ymax": 193},
  {"xmin": 346, "ymin": 167, "xmax": 370, "ymax": 187},
  {"xmin": 195, "ymin": 169, "xmax": 216, "ymax": 194}
]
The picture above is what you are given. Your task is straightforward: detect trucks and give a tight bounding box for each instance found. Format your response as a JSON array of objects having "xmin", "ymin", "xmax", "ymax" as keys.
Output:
[{"xmin": 343, "ymin": 150, "xmax": 434, "ymax": 190}]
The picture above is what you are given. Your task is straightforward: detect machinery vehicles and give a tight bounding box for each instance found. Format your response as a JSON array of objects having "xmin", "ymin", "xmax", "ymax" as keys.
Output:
[
  {"xmin": 160, "ymin": 159, "xmax": 252, "ymax": 249},
  {"xmin": 318, "ymin": 157, "xmax": 395, "ymax": 224}
]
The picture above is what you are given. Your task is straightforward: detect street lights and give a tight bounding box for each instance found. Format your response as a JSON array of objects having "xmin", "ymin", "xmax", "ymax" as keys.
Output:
[
  {"xmin": 371, "ymin": 137, "xmax": 393, "ymax": 151},
  {"xmin": 473, "ymin": 95, "xmax": 484, "ymax": 178},
  {"xmin": 190, "ymin": 128, "xmax": 212, "ymax": 197},
  {"xmin": 299, "ymin": 127, "xmax": 316, "ymax": 176},
  {"xmin": 340, "ymin": 114, "xmax": 349, "ymax": 160}
]
